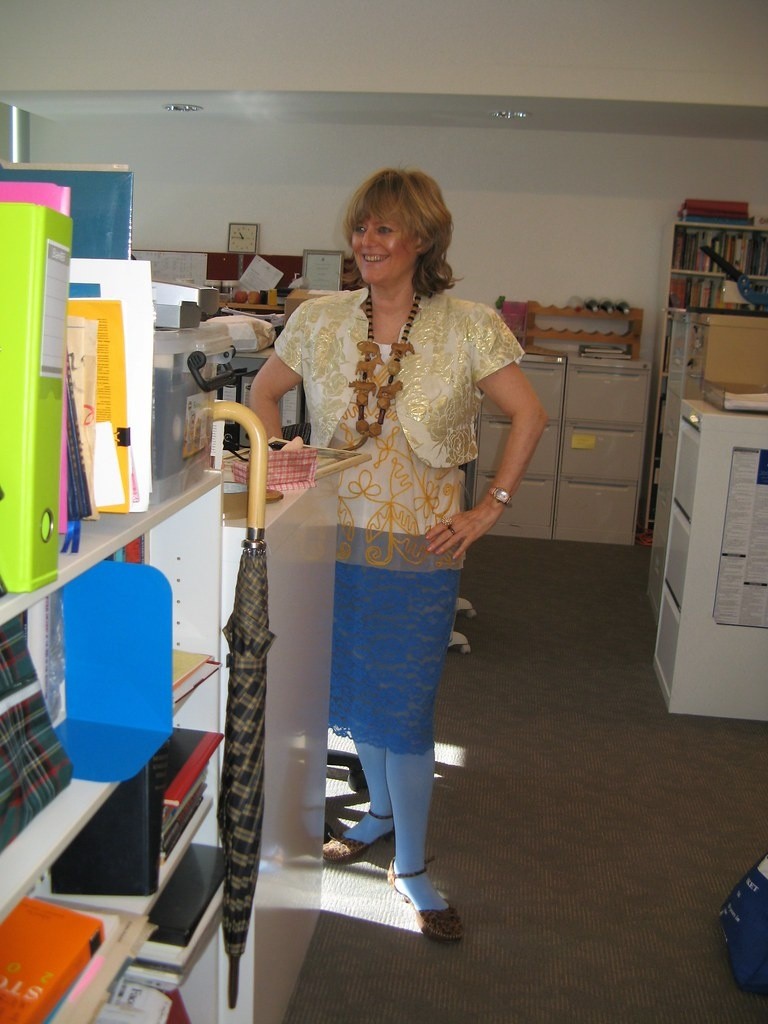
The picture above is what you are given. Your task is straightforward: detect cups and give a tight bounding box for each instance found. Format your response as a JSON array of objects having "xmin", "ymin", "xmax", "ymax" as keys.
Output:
[
  {"xmin": 222, "ymin": 280, "xmax": 239, "ymax": 302},
  {"xmin": 205, "ymin": 280, "xmax": 221, "ymax": 292}
]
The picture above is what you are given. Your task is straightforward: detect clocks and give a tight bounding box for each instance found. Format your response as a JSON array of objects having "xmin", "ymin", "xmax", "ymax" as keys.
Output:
[{"xmin": 227, "ymin": 223, "xmax": 258, "ymax": 252}]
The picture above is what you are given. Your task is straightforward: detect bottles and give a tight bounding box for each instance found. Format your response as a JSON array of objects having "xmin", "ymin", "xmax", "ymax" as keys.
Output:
[
  {"xmin": 614, "ymin": 298, "xmax": 630, "ymax": 317},
  {"xmin": 585, "ymin": 297, "xmax": 598, "ymax": 312},
  {"xmin": 598, "ymin": 296, "xmax": 613, "ymax": 315},
  {"xmin": 571, "ymin": 296, "xmax": 584, "ymax": 312}
]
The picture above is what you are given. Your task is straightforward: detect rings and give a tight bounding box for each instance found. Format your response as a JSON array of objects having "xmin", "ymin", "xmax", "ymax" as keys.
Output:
[{"xmin": 441, "ymin": 513, "xmax": 456, "ymax": 535}]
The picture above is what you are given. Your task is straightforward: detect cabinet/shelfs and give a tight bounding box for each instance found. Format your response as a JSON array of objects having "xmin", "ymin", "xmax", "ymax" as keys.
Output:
[
  {"xmin": 472, "ymin": 353, "xmax": 568, "ymax": 539},
  {"xmin": 652, "ymin": 396, "xmax": 768, "ymax": 721},
  {"xmin": 500, "ymin": 301, "xmax": 644, "ymax": 361},
  {"xmin": 0, "ymin": 464, "xmax": 230, "ymax": 1024},
  {"xmin": 642, "ymin": 220, "xmax": 768, "ymax": 535},
  {"xmin": 551, "ymin": 352, "xmax": 654, "ymax": 546}
]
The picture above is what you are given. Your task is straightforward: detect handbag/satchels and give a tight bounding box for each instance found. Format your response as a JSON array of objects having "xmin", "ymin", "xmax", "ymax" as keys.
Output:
[{"xmin": 718, "ymin": 856, "xmax": 768, "ymax": 992}]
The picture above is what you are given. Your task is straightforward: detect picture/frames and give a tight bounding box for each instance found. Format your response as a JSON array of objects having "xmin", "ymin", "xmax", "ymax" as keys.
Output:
[{"xmin": 303, "ymin": 248, "xmax": 344, "ymax": 292}]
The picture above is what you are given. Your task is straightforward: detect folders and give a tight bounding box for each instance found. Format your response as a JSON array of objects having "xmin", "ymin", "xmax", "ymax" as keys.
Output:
[{"xmin": 0, "ymin": 201, "xmax": 74, "ymax": 594}]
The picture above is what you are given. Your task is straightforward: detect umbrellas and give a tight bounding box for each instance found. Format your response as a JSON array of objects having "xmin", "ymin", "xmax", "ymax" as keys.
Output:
[{"xmin": 190, "ymin": 394, "xmax": 274, "ymax": 1009}]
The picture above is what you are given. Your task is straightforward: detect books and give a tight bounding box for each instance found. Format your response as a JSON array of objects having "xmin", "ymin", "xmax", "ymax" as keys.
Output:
[
  {"xmin": 0, "ymin": 157, "xmax": 154, "ymax": 603},
  {"xmin": 669, "ymin": 198, "xmax": 768, "ymax": 312},
  {"xmin": 0, "ymin": 728, "xmax": 225, "ymax": 1024}
]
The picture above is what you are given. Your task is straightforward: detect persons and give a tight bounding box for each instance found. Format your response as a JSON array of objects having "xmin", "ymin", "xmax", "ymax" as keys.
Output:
[{"xmin": 250, "ymin": 167, "xmax": 549, "ymax": 945}]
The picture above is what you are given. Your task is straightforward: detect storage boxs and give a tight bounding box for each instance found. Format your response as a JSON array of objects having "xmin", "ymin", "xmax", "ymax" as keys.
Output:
[
  {"xmin": 685, "ymin": 312, "xmax": 768, "ymax": 385},
  {"xmin": 148, "ymin": 322, "xmax": 234, "ymax": 506}
]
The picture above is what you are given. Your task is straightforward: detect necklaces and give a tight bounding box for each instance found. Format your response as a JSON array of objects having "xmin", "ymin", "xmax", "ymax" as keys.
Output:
[{"xmin": 356, "ymin": 285, "xmax": 422, "ymax": 434}]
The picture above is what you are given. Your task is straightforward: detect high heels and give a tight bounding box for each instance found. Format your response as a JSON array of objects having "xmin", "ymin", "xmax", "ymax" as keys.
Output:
[
  {"xmin": 323, "ymin": 810, "xmax": 393, "ymax": 861},
  {"xmin": 388, "ymin": 857, "xmax": 464, "ymax": 943}
]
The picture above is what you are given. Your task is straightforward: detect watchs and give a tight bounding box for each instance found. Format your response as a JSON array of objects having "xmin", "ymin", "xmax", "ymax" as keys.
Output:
[{"xmin": 488, "ymin": 486, "xmax": 513, "ymax": 508}]
